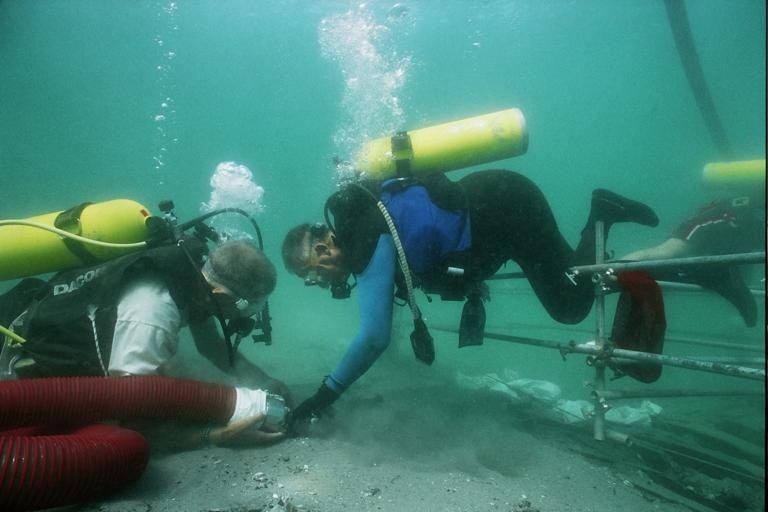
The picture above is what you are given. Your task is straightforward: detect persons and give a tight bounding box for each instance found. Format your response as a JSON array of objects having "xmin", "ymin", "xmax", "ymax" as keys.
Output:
[
  {"xmin": 1, "ymin": 224, "xmax": 297, "ymax": 447},
  {"xmin": 280, "ymin": 166, "xmax": 757, "ymax": 435}
]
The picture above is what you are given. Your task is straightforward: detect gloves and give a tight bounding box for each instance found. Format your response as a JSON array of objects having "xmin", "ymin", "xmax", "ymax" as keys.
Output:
[{"xmin": 287, "ymin": 387, "xmax": 345, "ymax": 425}]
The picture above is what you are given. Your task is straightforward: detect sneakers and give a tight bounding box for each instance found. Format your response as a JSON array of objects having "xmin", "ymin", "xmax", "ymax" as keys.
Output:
[
  {"xmin": 703, "ymin": 262, "xmax": 757, "ymax": 327},
  {"xmin": 584, "ymin": 188, "xmax": 660, "ymax": 236}
]
